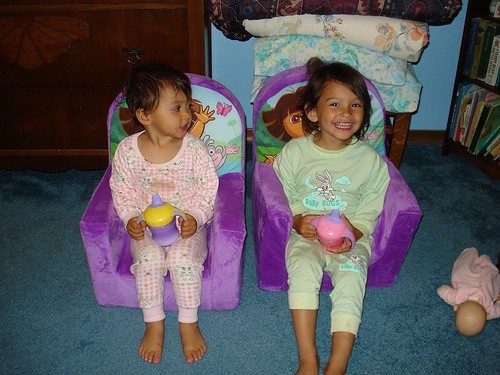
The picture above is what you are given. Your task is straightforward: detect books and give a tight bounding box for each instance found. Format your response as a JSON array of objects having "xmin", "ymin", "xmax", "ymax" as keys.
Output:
[{"xmin": 448, "ymin": 17, "xmax": 500, "ymax": 161}]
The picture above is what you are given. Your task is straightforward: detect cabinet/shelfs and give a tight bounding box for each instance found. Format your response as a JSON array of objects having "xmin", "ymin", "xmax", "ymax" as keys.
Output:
[
  {"xmin": 442, "ymin": 0, "xmax": 500, "ymax": 182},
  {"xmin": 0, "ymin": 0, "xmax": 213, "ymax": 173}
]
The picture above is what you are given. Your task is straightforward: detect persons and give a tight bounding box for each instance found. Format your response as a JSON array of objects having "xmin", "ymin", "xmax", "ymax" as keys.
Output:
[
  {"xmin": 436, "ymin": 246, "xmax": 500, "ymax": 337},
  {"xmin": 272, "ymin": 57, "xmax": 390, "ymax": 375},
  {"xmin": 109, "ymin": 64, "xmax": 219, "ymax": 362}
]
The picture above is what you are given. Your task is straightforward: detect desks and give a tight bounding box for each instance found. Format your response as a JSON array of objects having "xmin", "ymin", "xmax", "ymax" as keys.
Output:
[{"xmin": 249, "ymin": 101, "xmax": 412, "ymax": 171}]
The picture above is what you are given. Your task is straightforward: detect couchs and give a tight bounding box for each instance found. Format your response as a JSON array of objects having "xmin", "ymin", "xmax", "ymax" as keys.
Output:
[
  {"xmin": 251, "ymin": 65, "xmax": 423, "ymax": 292},
  {"xmin": 79, "ymin": 72, "xmax": 247, "ymax": 312}
]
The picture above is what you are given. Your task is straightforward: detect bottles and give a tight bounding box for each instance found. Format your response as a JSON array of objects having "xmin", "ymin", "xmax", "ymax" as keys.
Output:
[
  {"xmin": 137, "ymin": 194, "xmax": 188, "ymax": 248},
  {"xmin": 312, "ymin": 208, "xmax": 355, "ymax": 254}
]
